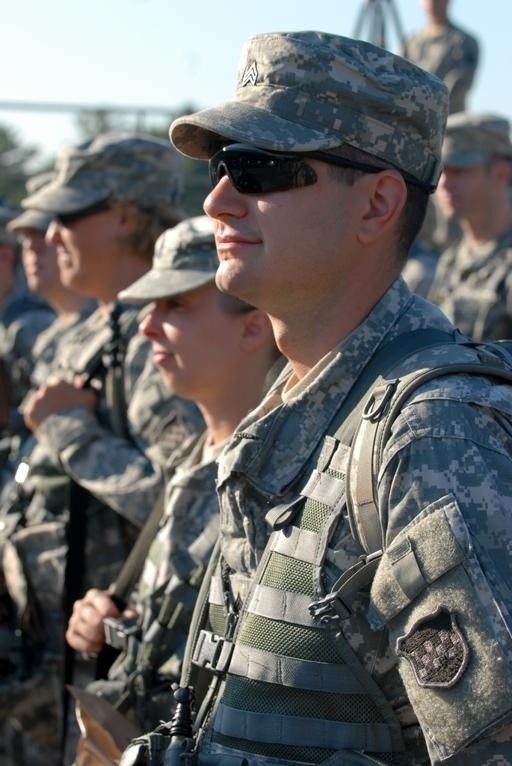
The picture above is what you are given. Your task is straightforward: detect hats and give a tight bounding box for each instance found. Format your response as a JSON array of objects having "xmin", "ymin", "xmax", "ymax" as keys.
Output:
[
  {"xmin": 7, "ymin": 133, "xmax": 180, "ymax": 231},
  {"xmin": 442, "ymin": 113, "xmax": 512, "ymax": 168},
  {"xmin": 117, "ymin": 216, "xmax": 220, "ymax": 302},
  {"xmin": 170, "ymin": 31, "xmax": 450, "ymax": 190}
]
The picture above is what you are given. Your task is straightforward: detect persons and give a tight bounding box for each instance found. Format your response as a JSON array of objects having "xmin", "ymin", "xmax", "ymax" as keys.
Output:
[{"xmin": 0, "ymin": 1, "xmax": 512, "ymax": 766}]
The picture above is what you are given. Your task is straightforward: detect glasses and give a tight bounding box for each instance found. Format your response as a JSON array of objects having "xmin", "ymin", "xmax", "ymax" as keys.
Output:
[{"xmin": 207, "ymin": 143, "xmax": 385, "ymax": 195}]
